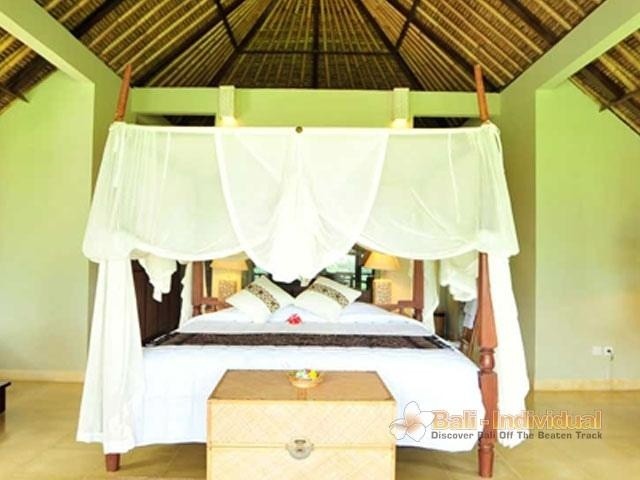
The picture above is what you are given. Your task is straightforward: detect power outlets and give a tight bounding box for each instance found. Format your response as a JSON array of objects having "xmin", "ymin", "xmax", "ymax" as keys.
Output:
[{"xmin": 604, "ymin": 345, "xmax": 612, "ymax": 357}]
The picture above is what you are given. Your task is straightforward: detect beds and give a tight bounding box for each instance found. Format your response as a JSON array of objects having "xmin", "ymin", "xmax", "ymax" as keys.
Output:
[{"xmin": 76, "ymin": 64, "xmax": 529, "ymax": 478}]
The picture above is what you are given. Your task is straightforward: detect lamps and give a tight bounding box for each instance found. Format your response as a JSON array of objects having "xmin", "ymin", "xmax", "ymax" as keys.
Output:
[
  {"xmin": 362, "ymin": 249, "xmax": 403, "ymax": 305},
  {"xmin": 218, "ymin": 83, "xmax": 237, "ymax": 121},
  {"xmin": 207, "ymin": 256, "xmax": 250, "ymax": 303},
  {"xmin": 391, "ymin": 86, "xmax": 410, "ymax": 123}
]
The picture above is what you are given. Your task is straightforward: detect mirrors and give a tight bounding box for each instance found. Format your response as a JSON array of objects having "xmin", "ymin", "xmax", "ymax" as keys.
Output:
[{"xmin": 252, "ymin": 252, "xmax": 374, "ymax": 290}]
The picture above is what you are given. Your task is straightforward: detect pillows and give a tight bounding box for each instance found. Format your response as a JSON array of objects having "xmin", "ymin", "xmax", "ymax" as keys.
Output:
[
  {"xmin": 295, "ymin": 276, "xmax": 362, "ymax": 323},
  {"xmin": 266, "ymin": 269, "xmax": 349, "ymax": 298},
  {"xmin": 224, "ymin": 274, "xmax": 295, "ymax": 321}
]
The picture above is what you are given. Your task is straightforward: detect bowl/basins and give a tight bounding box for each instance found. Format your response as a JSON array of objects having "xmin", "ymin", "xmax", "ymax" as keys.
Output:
[{"xmin": 289, "ymin": 376, "xmax": 322, "ymax": 389}]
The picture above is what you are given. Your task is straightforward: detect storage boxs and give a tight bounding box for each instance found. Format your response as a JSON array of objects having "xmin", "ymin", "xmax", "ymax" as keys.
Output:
[{"xmin": 206, "ymin": 369, "xmax": 396, "ymax": 477}]
